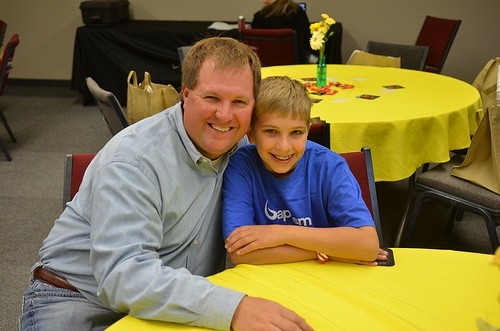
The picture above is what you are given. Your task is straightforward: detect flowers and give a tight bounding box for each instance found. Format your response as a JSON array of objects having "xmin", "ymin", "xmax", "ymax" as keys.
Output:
[{"xmin": 309, "ymin": 13, "xmax": 336, "ymax": 57}]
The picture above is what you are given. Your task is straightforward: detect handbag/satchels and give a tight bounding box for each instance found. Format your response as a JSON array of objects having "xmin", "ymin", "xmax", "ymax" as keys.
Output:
[{"xmin": 126, "ymin": 71, "xmax": 178, "ymax": 125}]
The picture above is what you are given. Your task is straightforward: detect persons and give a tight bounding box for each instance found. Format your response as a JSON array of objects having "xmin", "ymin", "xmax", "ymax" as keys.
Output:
[
  {"xmin": 221, "ymin": 76, "xmax": 388, "ymax": 266},
  {"xmin": 252, "ymin": 0, "xmax": 311, "ymax": 63},
  {"xmin": 18, "ymin": 36, "xmax": 315, "ymax": 331}
]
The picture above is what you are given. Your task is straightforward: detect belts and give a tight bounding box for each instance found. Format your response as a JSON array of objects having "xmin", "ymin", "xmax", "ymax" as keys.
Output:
[{"xmin": 32, "ymin": 267, "xmax": 82, "ymax": 293}]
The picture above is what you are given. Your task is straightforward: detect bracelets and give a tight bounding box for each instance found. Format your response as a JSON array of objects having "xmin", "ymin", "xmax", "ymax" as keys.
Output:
[{"xmin": 316, "ymin": 251, "xmax": 328, "ymax": 264}]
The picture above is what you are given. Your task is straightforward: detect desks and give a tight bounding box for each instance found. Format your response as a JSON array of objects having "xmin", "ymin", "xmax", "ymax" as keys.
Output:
[
  {"xmin": 104, "ymin": 246, "xmax": 500, "ymax": 331},
  {"xmin": 259, "ymin": 64, "xmax": 485, "ymax": 182},
  {"xmin": 69, "ymin": 19, "xmax": 343, "ymax": 109}
]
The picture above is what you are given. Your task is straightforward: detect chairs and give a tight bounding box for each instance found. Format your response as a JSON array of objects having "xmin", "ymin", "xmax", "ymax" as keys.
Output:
[
  {"xmin": 237, "ymin": 15, "xmax": 298, "ymax": 68},
  {"xmin": 63, "ymin": 117, "xmax": 384, "ymax": 249},
  {"xmin": 390, "ymin": 55, "xmax": 500, "ymax": 256},
  {"xmin": 86, "ymin": 77, "xmax": 133, "ymax": 138},
  {"xmin": 344, "ymin": 13, "xmax": 462, "ymax": 74},
  {"xmin": 0, "ymin": 18, "xmax": 20, "ymax": 161}
]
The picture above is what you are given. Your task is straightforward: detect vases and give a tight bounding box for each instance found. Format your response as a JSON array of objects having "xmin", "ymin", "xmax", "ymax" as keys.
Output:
[{"xmin": 317, "ymin": 54, "xmax": 326, "ymax": 88}]
